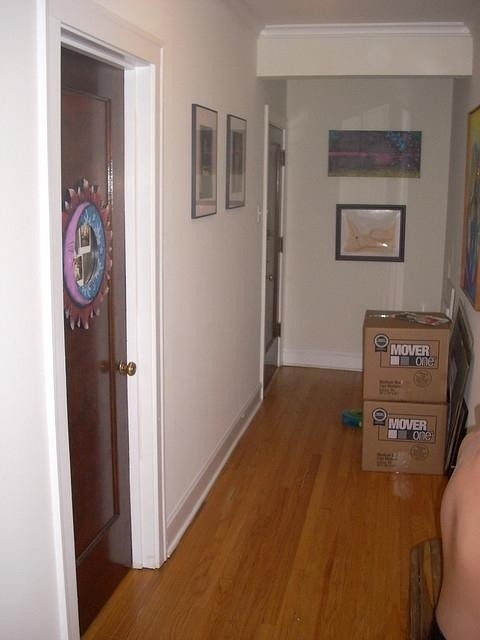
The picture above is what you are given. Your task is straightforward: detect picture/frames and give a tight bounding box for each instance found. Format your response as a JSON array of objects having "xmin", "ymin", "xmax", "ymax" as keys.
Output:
[
  {"xmin": 460, "ymin": 104, "xmax": 480, "ymax": 312},
  {"xmin": 335, "ymin": 204, "xmax": 406, "ymax": 262},
  {"xmin": 192, "ymin": 104, "xmax": 217, "ymax": 219},
  {"xmin": 226, "ymin": 114, "xmax": 247, "ymax": 209}
]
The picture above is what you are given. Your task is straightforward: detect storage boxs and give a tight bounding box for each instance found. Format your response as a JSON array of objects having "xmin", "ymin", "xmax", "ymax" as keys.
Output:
[
  {"xmin": 362, "ymin": 401, "xmax": 448, "ymax": 475},
  {"xmin": 362, "ymin": 310, "xmax": 452, "ymax": 404}
]
